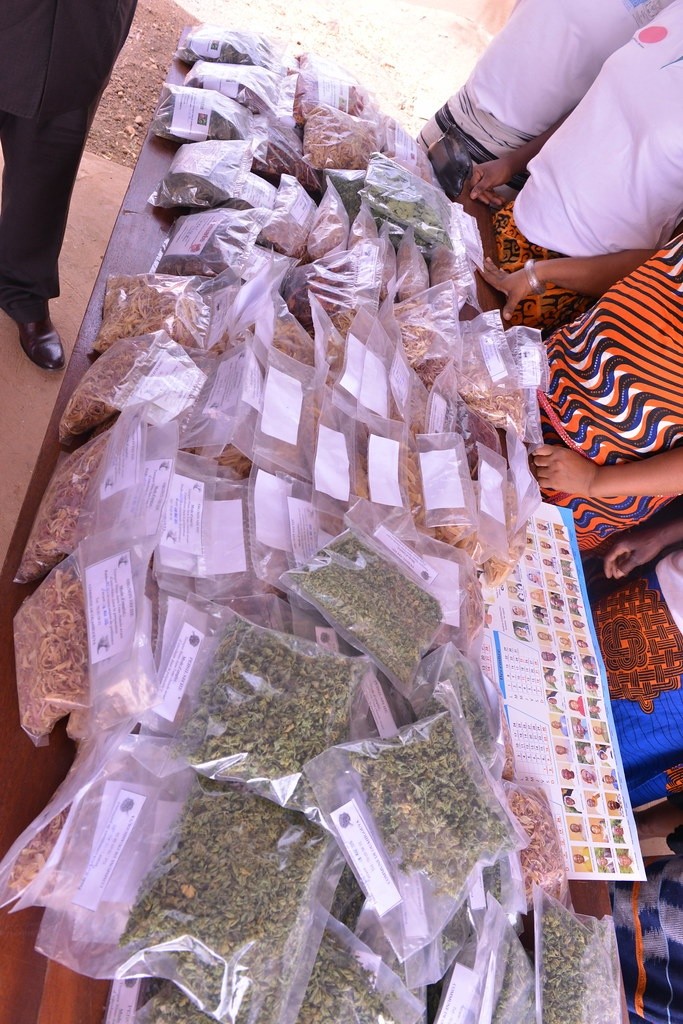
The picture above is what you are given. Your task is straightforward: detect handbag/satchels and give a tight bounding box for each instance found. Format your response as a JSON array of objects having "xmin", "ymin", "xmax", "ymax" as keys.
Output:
[{"xmin": 427, "ymin": 125, "xmax": 474, "ymax": 202}]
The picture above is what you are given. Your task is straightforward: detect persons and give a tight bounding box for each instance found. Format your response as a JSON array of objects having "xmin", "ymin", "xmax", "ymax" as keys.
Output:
[
  {"xmin": 587, "ymin": 519, "xmax": 683, "ymax": 1024},
  {"xmin": 0, "ymin": 1, "xmax": 137, "ymax": 370},
  {"xmin": 474, "ymin": 230, "xmax": 683, "ymax": 551},
  {"xmin": 415, "ymin": 0, "xmax": 674, "ymax": 201},
  {"xmin": 469, "ymin": 0, "xmax": 683, "ymax": 335}
]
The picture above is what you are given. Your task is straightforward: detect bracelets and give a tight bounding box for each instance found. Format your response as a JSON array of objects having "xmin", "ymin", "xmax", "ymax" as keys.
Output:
[{"xmin": 525, "ymin": 259, "xmax": 545, "ymax": 295}]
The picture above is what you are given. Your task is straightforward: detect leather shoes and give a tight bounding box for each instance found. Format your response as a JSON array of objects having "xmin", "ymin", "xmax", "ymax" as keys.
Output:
[{"xmin": 12, "ymin": 316, "xmax": 66, "ymax": 372}]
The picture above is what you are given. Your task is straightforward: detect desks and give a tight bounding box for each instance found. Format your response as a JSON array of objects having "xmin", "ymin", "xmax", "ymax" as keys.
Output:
[{"xmin": 0, "ymin": 25, "xmax": 630, "ymax": 1024}]
